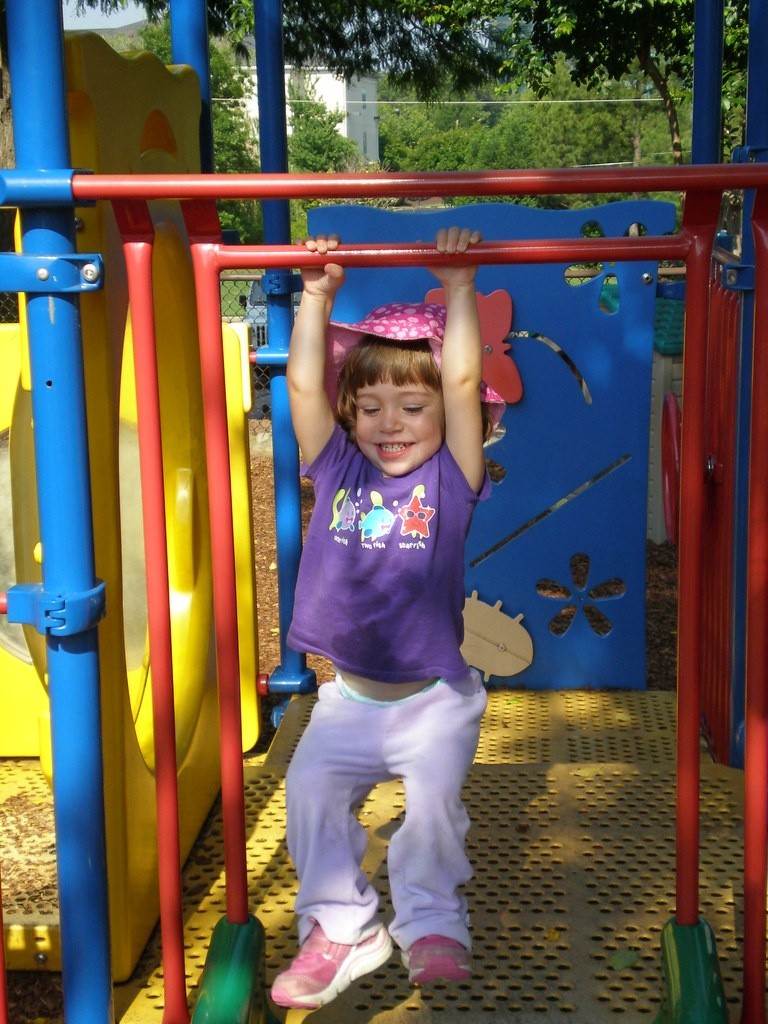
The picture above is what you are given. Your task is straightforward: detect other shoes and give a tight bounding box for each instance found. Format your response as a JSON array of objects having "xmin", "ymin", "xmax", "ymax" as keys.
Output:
[
  {"xmin": 399, "ymin": 934, "xmax": 474, "ymax": 986},
  {"xmin": 271, "ymin": 917, "xmax": 394, "ymax": 1009}
]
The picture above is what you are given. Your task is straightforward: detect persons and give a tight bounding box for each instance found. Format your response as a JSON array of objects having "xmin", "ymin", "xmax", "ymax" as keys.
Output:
[{"xmin": 268, "ymin": 225, "xmax": 489, "ymax": 1011}]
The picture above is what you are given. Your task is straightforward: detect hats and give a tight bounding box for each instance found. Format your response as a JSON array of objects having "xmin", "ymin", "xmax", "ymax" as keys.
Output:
[{"xmin": 323, "ymin": 302, "xmax": 507, "ymax": 433}]
